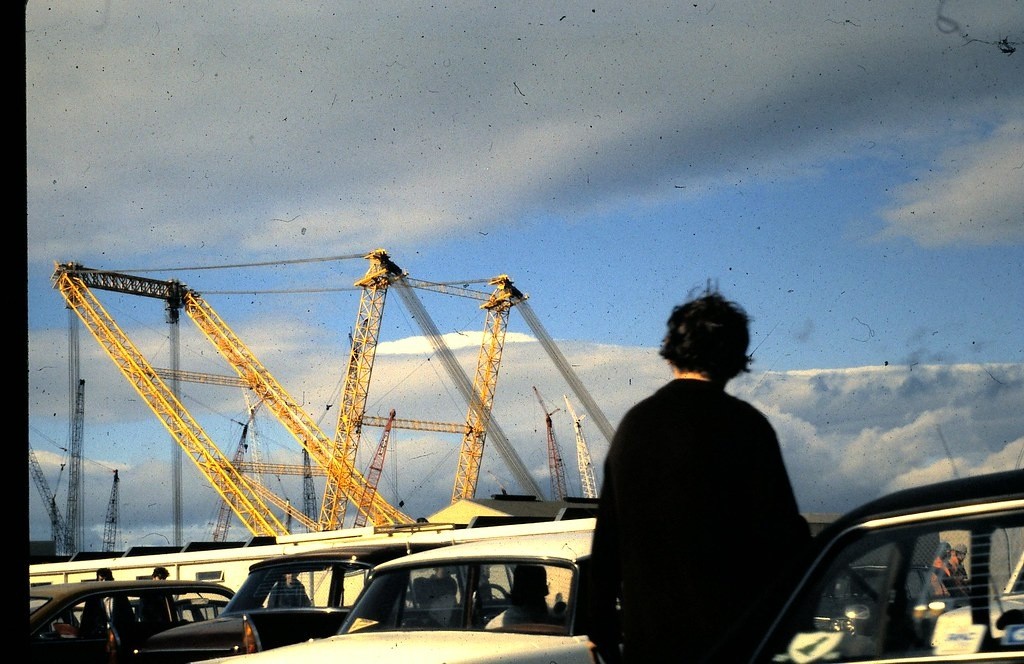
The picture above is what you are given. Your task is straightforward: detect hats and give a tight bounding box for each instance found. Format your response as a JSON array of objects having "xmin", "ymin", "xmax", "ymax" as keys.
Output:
[{"xmin": 951, "ymin": 543, "xmax": 967, "ymax": 554}]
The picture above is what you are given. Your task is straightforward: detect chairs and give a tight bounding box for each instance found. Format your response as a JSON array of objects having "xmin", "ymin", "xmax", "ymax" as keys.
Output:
[
  {"xmin": 410, "ymin": 576, "xmax": 457, "ymax": 608},
  {"xmin": 483, "ymin": 565, "xmax": 551, "ymax": 630}
]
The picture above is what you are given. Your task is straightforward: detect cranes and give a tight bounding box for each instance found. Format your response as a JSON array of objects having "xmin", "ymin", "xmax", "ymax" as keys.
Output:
[
  {"xmin": 301, "ymin": 363, "xmax": 346, "ymax": 532},
  {"xmin": 53, "ymin": 250, "xmax": 617, "ymax": 539},
  {"xmin": 215, "ymin": 423, "xmax": 249, "ymax": 543},
  {"xmin": 534, "ymin": 386, "xmax": 568, "ymax": 501},
  {"xmin": 563, "ymin": 393, "xmax": 598, "ymax": 498},
  {"xmin": 29, "ymin": 379, "xmax": 119, "ymax": 558},
  {"xmin": 353, "ymin": 409, "xmax": 398, "ymax": 528}
]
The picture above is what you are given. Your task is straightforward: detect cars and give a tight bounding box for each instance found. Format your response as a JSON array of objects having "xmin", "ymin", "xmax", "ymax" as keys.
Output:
[
  {"xmin": 193, "ymin": 542, "xmax": 612, "ymax": 664},
  {"xmin": 749, "ymin": 468, "xmax": 1023, "ymax": 664},
  {"xmin": 29, "ymin": 580, "xmax": 238, "ymax": 664},
  {"xmin": 133, "ymin": 541, "xmax": 518, "ymax": 664}
]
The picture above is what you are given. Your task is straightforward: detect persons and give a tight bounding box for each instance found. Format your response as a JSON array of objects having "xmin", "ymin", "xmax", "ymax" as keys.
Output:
[
  {"xmin": 584, "ymin": 280, "xmax": 824, "ymax": 664},
  {"xmin": 428, "ymin": 567, "xmax": 457, "ymax": 602},
  {"xmin": 485, "ymin": 566, "xmax": 552, "ymax": 630},
  {"xmin": 267, "ymin": 573, "xmax": 312, "ymax": 608},
  {"xmin": 929, "ymin": 542, "xmax": 970, "ymax": 596},
  {"xmin": 95, "ymin": 566, "xmax": 170, "ymax": 628}
]
